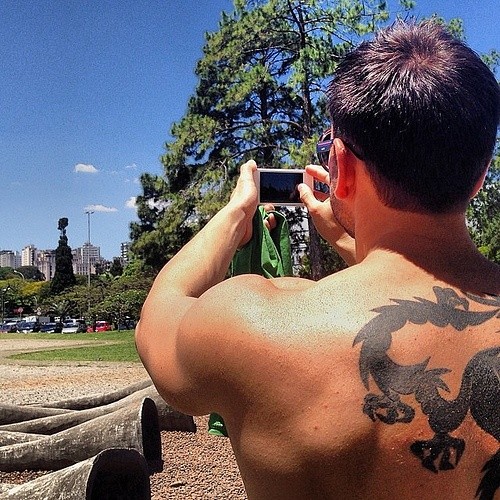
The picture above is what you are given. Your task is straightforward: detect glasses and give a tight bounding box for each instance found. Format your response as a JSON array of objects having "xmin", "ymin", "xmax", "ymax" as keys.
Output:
[{"xmin": 316, "ymin": 128, "xmax": 334, "ymax": 172}]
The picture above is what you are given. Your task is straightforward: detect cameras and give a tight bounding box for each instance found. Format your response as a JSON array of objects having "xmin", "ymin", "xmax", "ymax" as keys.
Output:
[{"xmin": 253, "ymin": 168, "xmax": 314, "ymax": 206}]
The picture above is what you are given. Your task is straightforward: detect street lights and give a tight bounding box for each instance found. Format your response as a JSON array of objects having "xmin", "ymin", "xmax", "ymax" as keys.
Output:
[
  {"xmin": 0, "ymin": 287, "xmax": 10, "ymax": 329},
  {"xmin": 84, "ymin": 211, "xmax": 95, "ymax": 309},
  {"xmin": 13, "ymin": 269, "xmax": 25, "ymax": 281}
]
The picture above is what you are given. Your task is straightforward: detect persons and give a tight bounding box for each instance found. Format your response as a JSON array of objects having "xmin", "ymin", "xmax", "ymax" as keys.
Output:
[{"xmin": 134, "ymin": 24, "xmax": 500, "ymax": 500}]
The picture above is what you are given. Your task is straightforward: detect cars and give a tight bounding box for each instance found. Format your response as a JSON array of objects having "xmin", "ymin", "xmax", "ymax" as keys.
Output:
[{"xmin": 0, "ymin": 315, "xmax": 139, "ymax": 334}]
